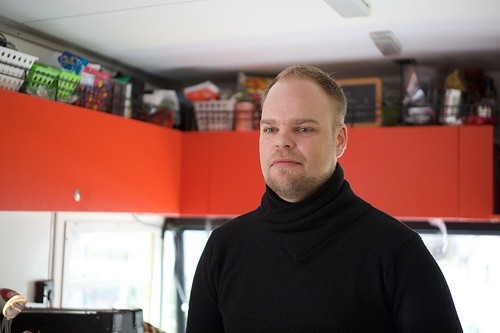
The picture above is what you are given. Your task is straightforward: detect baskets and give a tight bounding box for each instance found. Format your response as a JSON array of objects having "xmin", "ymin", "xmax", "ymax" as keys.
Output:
[
  {"xmin": 74, "ymin": 84, "xmax": 110, "ymax": 112},
  {"xmin": 0, "ymin": 46, "xmax": 39, "ymax": 92},
  {"xmin": 27, "ymin": 64, "xmax": 81, "ymax": 103},
  {"xmin": 193, "ymin": 100, "xmax": 235, "ymax": 130}
]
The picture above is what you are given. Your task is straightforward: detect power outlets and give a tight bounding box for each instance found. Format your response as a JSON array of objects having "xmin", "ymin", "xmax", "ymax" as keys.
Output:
[{"xmin": 35, "ymin": 280, "xmax": 53, "ymax": 303}]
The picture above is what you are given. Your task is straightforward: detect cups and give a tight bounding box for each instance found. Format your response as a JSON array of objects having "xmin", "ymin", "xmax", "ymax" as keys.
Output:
[
  {"xmin": 439, "ymin": 89, "xmax": 464, "ymax": 126},
  {"xmin": 235, "ymin": 101, "xmax": 256, "ymax": 130},
  {"xmin": 111, "ymin": 82, "xmax": 133, "ymax": 117}
]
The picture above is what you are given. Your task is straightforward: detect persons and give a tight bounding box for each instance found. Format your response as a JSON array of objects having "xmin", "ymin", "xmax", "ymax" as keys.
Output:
[{"xmin": 186, "ymin": 64, "xmax": 465, "ymax": 333}]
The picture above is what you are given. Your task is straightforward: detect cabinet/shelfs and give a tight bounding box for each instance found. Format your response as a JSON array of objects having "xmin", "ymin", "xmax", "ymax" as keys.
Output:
[{"xmin": 1, "ymin": 88, "xmax": 494, "ymax": 222}]
